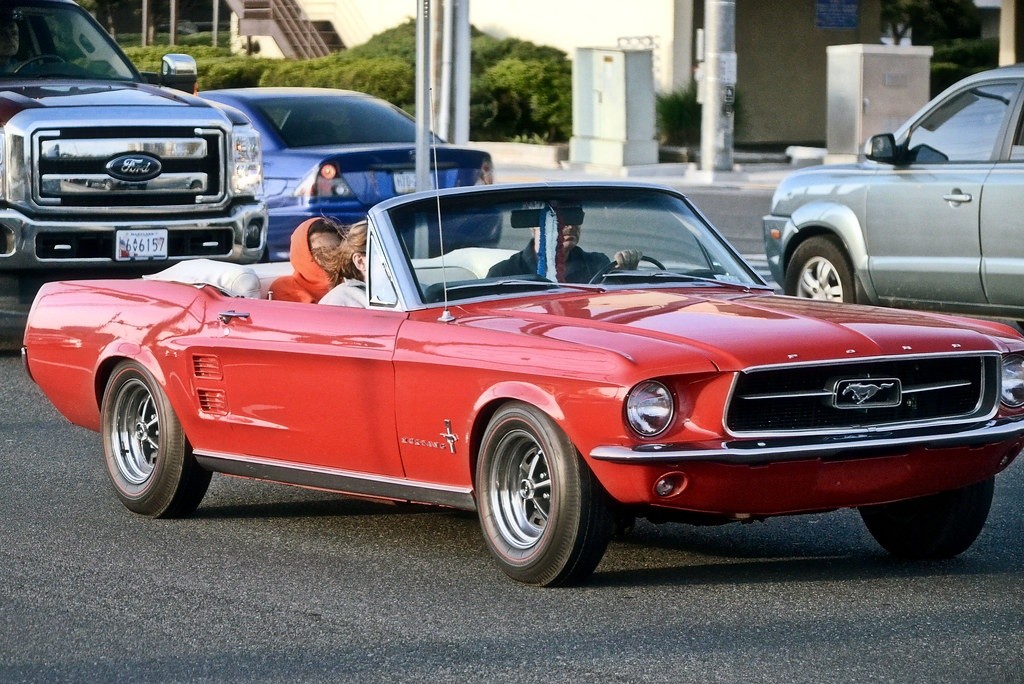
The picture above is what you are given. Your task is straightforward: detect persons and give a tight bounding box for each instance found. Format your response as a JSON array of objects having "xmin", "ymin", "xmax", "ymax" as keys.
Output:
[
  {"xmin": 318, "ymin": 220, "xmax": 369, "ymax": 308},
  {"xmin": 485, "ymin": 199, "xmax": 643, "ymax": 286},
  {"xmin": 0, "ymin": 18, "xmax": 41, "ymax": 73},
  {"xmin": 265, "ymin": 217, "xmax": 348, "ymax": 305}
]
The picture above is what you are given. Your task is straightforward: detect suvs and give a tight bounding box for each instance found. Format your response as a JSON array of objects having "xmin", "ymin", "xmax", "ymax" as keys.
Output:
[{"xmin": 1, "ymin": 0, "xmax": 270, "ymax": 354}]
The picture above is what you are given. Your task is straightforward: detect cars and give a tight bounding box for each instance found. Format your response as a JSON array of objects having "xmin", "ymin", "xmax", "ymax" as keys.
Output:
[
  {"xmin": 193, "ymin": 85, "xmax": 494, "ymax": 263},
  {"xmin": 761, "ymin": 60, "xmax": 1023, "ymax": 330}
]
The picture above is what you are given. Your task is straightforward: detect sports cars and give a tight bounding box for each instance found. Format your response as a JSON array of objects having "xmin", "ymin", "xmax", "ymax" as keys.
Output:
[{"xmin": 21, "ymin": 174, "xmax": 1023, "ymax": 591}]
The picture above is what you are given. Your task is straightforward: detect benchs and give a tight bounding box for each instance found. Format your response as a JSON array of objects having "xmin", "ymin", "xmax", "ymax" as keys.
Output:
[{"xmin": 259, "ymin": 265, "xmax": 477, "ymax": 298}]
